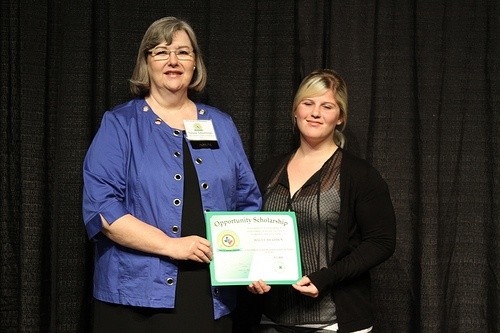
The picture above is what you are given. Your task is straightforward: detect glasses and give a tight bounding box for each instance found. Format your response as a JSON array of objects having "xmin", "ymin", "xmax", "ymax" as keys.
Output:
[{"xmin": 147, "ymin": 49, "xmax": 196, "ymax": 61}]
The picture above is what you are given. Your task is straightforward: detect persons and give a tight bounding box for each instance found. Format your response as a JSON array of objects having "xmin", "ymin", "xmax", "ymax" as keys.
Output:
[
  {"xmin": 242, "ymin": 67, "xmax": 399, "ymax": 333},
  {"xmin": 81, "ymin": 15, "xmax": 267, "ymax": 333}
]
